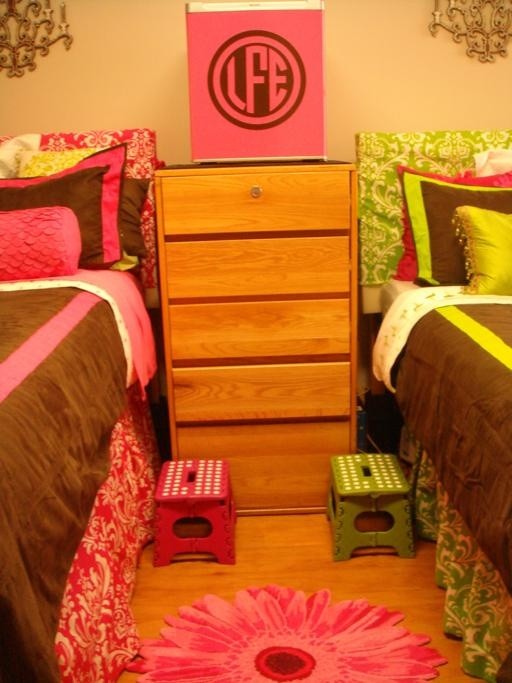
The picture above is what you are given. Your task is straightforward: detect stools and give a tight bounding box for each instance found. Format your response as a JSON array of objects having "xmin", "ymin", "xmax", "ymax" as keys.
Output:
[
  {"xmin": 324, "ymin": 452, "xmax": 416, "ymax": 565},
  {"xmin": 150, "ymin": 458, "xmax": 240, "ymax": 568}
]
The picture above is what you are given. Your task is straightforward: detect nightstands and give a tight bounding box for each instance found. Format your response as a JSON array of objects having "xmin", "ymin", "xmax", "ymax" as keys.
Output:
[{"xmin": 152, "ymin": 159, "xmax": 359, "ymax": 523}]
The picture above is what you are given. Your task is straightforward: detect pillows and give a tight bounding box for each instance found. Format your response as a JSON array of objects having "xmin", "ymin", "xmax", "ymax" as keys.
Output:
[
  {"xmin": 385, "ymin": 153, "xmax": 511, "ymax": 298},
  {"xmin": 0, "ymin": 142, "xmax": 155, "ymax": 285}
]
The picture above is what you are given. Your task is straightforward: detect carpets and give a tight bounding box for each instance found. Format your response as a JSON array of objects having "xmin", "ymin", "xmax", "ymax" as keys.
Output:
[{"xmin": 126, "ymin": 581, "xmax": 453, "ymax": 683}]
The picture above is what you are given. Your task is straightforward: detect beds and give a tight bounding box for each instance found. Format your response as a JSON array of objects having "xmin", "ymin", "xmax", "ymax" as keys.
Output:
[
  {"xmin": 353, "ymin": 127, "xmax": 512, "ymax": 683},
  {"xmin": 0, "ymin": 129, "xmax": 155, "ymax": 683}
]
